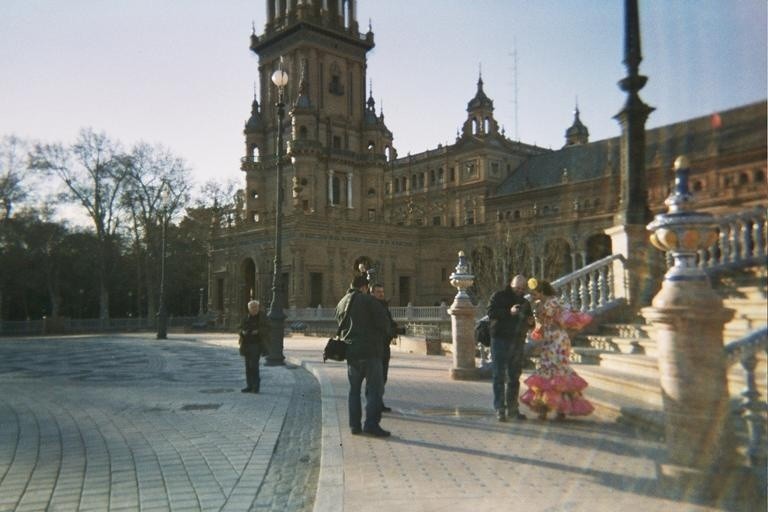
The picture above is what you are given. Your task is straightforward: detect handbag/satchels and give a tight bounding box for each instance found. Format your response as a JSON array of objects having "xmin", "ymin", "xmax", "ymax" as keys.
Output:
[
  {"xmin": 474, "ymin": 321, "xmax": 491, "ymax": 347},
  {"xmin": 323, "ymin": 337, "xmax": 346, "ymax": 364}
]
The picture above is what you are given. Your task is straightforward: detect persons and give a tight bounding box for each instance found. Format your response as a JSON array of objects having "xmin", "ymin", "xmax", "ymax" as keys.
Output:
[
  {"xmin": 488, "ymin": 273, "xmax": 535, "ymax": 420},
  {"xmin": 335, "ymin": 276, "xmax": 393, "ymax": 437},
  {"xmin": 440, "ymin": 299, "xmax": 447, "ymax": 307},
  {"xmin": 364, "ymin": 283, "xmax": 398, "ymax": 413},
  {"xmin": 238, "ymin": 301, "xmax": 271, "ymax": 393},
  {"xmin": 519, "ymin": 282, "xmax": 595, "ymax": 423}
]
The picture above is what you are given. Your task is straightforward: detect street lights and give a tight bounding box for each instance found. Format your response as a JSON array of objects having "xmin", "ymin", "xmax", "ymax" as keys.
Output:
[
  {"xmin": 266, "ymin": 55, "xmax": 290, "ymax": 366},
  {"xmin": 156, "ymin": 181, "xmax": 170, "ymax": 340}
]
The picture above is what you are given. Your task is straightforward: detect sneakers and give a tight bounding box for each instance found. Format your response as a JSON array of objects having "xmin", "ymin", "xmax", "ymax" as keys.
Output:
[
  {"xmin": 496, "ymin": 411, "xmax": 528, "ymax": 423},
  {"xmin": 350, "ymin": 423, "xmax": 391, "ymax": 437},
  {"xmin": 537, "ymin": 412, "xmax": 566, "ymax": 422},
  {"xmin": 380, "ymin": 406, "xmax": 392, "ymax": 413},
  {"xmin": 241, "ymin": 386, "xmax": 259, "ymax": 393}
]
